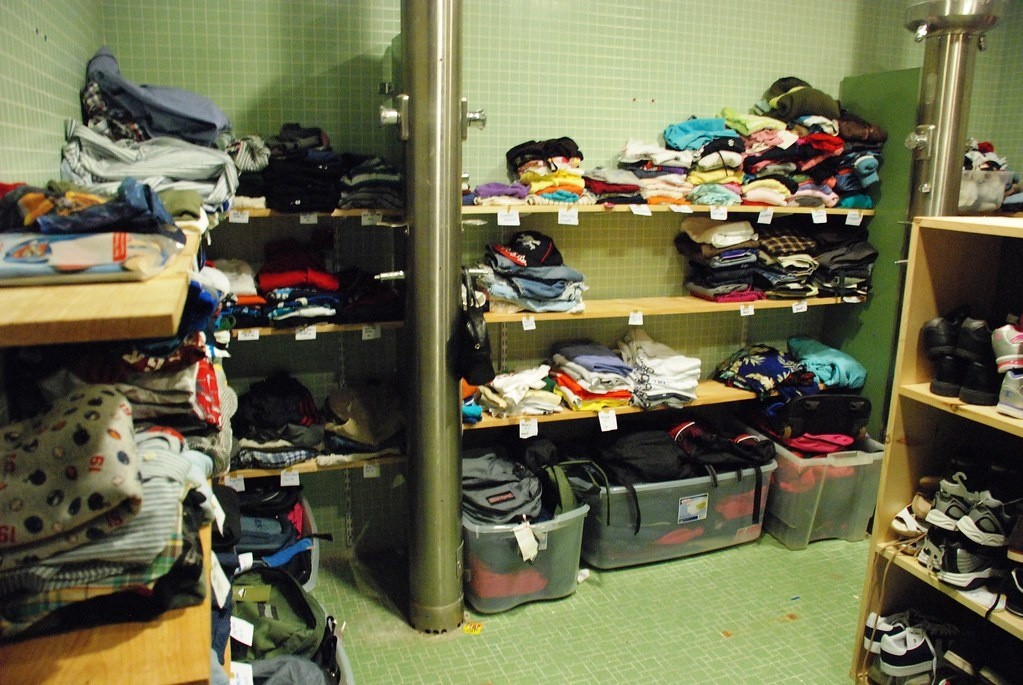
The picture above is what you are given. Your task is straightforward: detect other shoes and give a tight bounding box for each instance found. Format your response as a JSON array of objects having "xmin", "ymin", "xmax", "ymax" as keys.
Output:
[{"xmin": 862, "ymin": 309, "xmax": 1023, "ymax": 684}]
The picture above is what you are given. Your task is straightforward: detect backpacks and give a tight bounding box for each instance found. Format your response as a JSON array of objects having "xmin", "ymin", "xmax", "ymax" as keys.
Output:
[
  {"xmin": 230, "ymin": 477, "xmax": 338, "ymax": 684},
  {"xmin": 461, "ymin": 385, "xmax": 872, "ymax": 609}
]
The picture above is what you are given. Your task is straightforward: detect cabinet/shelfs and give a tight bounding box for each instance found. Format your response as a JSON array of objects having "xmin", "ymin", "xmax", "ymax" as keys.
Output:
[
  {"xmin": 452, "ymin": 176, "xmax": 875, "ymax": 440},
  {"xmin": 0, "ymin": 132, "xmax": 419, "ymax": 685},
  {"xmin": 848, "ymin": 196, "xmax": 1023, "ymax": 683}
]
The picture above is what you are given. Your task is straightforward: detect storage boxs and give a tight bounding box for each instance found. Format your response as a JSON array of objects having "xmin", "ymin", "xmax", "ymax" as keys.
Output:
[
  {"xmin": 957, "ymin": 166, "xmax": 1012, "ymax": 214},
  {"xmin": 562, "ymin": 449, "xmax": 777, "ymax": 569},
  {"xmin": 736, "ymin": 422, "xmax": 889, "ymax": 552},
  {"xmin": 462, "ymin": 500, "xmax": 591, "ymax": 617}
]
